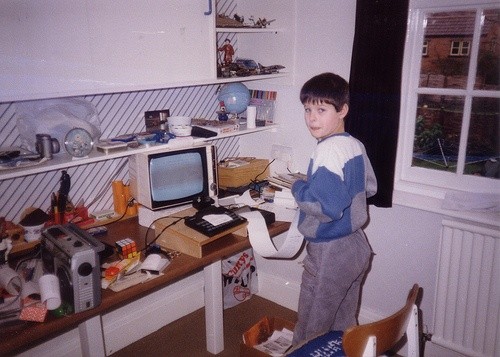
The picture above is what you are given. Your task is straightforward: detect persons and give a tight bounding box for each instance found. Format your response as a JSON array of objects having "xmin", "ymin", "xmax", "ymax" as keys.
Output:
[
  {"xmin": 291, "ymin": 72, "xmax": 378, "ymax": 347},
  {"xmin": 218, "ymin": 39, "xmax": 234, "ymax": 67}
]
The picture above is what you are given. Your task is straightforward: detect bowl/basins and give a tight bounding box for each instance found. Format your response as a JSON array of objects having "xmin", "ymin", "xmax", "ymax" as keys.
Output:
[
  {"xmin": 19, "ymin": 221, "xmax": 46, "ymax": 242},
  {"xmin": 167, "ymin": 117, "xmax": 191, "ymax": 134},
  {"xmin": 173, "ymin": 126, "xmax": 192, "ymax": 136}
]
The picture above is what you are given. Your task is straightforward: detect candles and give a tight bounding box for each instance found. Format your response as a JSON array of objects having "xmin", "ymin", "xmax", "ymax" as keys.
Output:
[{"xmin": 247, "ymin": 106, "xmax": 257, "ymax": 129}]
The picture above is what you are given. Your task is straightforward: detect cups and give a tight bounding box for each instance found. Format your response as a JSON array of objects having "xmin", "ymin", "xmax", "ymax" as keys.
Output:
[{"xmin": 35, "ymin": 134, "xmax": 53, "ymax": 161}]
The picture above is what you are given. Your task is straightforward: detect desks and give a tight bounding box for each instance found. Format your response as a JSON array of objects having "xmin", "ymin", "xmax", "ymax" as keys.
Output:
[{"xmin": 0, "ymin": 191, "xmax": 290, "ymax": 357}]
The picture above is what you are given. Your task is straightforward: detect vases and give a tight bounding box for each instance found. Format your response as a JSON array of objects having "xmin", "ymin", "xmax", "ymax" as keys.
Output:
[{"xmin": 19, "ymin": 222, "xmax": 46, "ymax": 243}]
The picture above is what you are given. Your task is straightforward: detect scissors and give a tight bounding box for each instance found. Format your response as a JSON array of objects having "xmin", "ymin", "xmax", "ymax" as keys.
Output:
[{"xmin": 146, "ymin": 243, "xmax": 182, "ymax": 259}]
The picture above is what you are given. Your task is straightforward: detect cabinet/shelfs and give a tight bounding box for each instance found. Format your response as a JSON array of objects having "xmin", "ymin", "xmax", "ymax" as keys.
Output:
[{"xmin": 0, "ymin": 0, "xmax": 289, "ymax": 181}]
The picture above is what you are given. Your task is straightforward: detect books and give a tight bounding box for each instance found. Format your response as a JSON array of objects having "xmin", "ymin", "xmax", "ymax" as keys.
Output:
[{"xmin": 267, "ymin": 172, "xmax": 307, "ymax": 190}]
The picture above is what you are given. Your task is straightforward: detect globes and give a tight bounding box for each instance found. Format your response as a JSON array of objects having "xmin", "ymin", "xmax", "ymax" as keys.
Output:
[{"xmin": 218, "ymin": 82, "xmax": 250, "ymax": 125}]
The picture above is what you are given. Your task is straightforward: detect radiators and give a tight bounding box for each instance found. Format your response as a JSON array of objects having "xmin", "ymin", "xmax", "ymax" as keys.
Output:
[{"xmin": 430, "ymin": 220, "xmax": 500, "ymax": 357}]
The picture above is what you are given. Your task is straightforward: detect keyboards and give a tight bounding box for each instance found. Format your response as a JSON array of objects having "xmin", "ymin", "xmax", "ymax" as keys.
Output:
[{"xmin": 184, "ymin": 205, "xmax": 248, "ymax": 237}]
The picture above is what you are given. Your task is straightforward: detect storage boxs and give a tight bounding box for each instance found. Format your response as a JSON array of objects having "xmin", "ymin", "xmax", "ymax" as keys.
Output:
[
  {"xmin": 219, "ymin": 158, "xmax": 270, "ymax": 188},
  {"xmin": 96, "ymin": 144, "xmax": 128, "ymax": 154},
  {"xmin": 240, "ymin": 316, "xmax": 297, "ymax": 357},
  {"xmin": 19, "ymin": 297, "xmax": 48, "ymax": 321}
]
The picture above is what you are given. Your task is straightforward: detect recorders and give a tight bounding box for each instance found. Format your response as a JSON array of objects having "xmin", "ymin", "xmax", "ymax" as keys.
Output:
[{"xmin": 40, "ymin": 223, "xmax": 106, "ymax": 313}]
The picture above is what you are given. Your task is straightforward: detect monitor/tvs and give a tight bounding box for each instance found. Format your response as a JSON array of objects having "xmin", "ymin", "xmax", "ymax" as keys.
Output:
[{"xmin": 128, "ymin": 142, "xmax": 219, "ymax": 210}]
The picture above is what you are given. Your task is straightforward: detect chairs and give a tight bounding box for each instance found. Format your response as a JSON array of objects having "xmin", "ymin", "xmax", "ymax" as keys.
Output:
[{"xmin": 287, "ymin": 283, "xmax": 420, "ymax": 357}]
